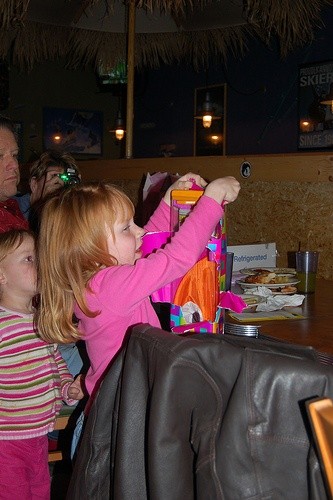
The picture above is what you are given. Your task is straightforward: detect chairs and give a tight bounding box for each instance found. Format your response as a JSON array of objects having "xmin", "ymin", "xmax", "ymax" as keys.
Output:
[{"xmin": 47, "ymin": 322, "xmax": 333, "ymax": 500}]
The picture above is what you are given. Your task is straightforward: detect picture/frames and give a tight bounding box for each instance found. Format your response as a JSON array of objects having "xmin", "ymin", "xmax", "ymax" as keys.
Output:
[
  {"xmin": 194, "ymin": 82, "xmax": 227, "ymax": 156},
  {"xmin": 42, "ymin": 106, "xmax": 104, "ymax": 156}
]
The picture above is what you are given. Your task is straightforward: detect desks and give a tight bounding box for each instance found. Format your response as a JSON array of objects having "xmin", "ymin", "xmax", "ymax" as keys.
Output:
[{"xmin": 225, "ymin": 273, "xmax": 333, "ymax": 359}]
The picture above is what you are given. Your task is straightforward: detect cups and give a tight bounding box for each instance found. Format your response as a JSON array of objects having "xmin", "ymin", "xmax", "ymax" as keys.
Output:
[
  {"xmin": 226, "ymin": 252, "xmax": 234, "ymax": 292},
  {"xmin": 296, "ymin": 250, "xmax": 319, "ymax": 294}
]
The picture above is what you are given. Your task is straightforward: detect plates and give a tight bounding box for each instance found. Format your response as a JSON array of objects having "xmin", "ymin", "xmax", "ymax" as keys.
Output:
[
  {"xmin": 240, "ymin": 267, "xmax": 296, "ymax": 277},
  {"xmin": 236, "ymin": 294, "xmax": 267, "ymax": 309},
  {"xmin": 244, "ymin": 286, "xmax": 297, "ymax": 295},
  {"xmin": 235, "ymin": 278, "xmax": 301, "ymax": 289}
]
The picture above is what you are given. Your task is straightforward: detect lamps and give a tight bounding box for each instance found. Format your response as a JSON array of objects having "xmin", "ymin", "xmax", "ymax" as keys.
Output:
[
  {"xmin": 193, "ymin": 66, "xmax": 222, "ymax": 128},
  {"xmin": 108, "ymin": 93, "xmax": 125, "ymax": 141},
  {"xmin": 320, "ymin": 83, "xmax": 333, "ymax": 114}
]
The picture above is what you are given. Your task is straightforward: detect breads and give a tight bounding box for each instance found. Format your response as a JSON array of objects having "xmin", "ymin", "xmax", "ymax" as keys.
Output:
[{"xmin": 246, "ymin": 273, "xmax": 276, "ymax": 283}]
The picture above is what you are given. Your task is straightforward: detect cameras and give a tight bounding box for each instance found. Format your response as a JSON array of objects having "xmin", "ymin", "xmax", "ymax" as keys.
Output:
[{"xmin": 56, "ymin": 168, "xmax": 81, "ymax": 186}]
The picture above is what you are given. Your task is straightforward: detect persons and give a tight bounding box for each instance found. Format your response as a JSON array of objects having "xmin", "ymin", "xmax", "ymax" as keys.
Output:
[
  {"xmin": 34, "ymin": 171, "xmax": 241, "ymax": 461},
  {"xmin": 0, "ymin": 117, "xmax": 88, "ymax": 500}
]
